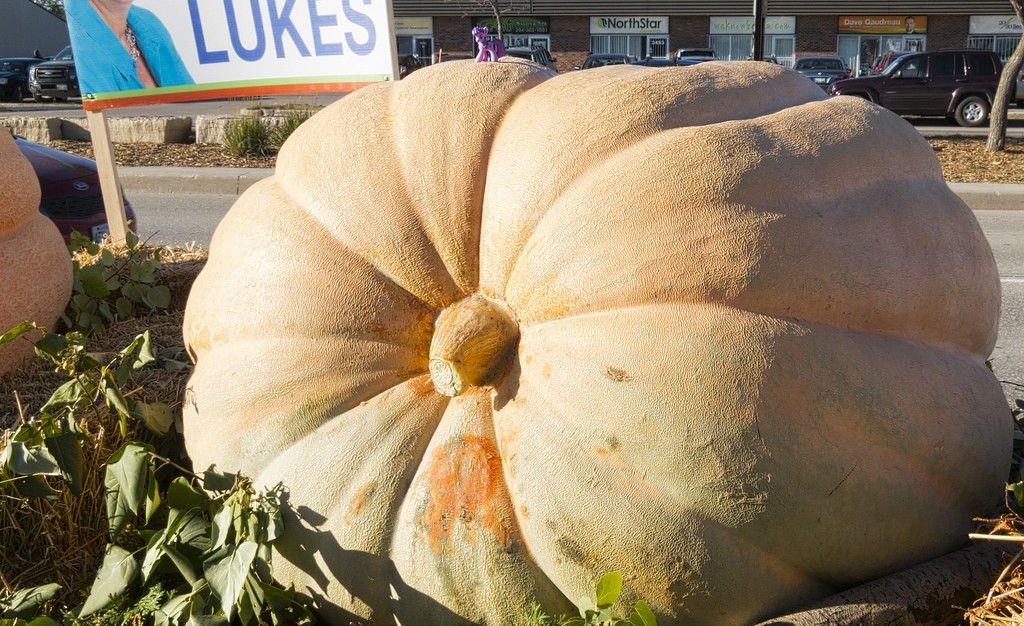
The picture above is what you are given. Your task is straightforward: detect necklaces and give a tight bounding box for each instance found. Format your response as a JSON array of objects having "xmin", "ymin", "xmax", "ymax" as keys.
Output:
[{"xmin": 123, "ymin": 25, "xmax": 141, "ymax": 62}]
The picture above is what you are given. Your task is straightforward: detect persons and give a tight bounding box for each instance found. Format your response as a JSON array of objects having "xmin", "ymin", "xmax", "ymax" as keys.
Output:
[
  {"xmin": 33, "ymin": 48, "xmax": 44, "ymax": 60},
  {"xmin": 903, "ymin": 17, "xmax": 919, "ymax": 34},
  {"xmin": 63, "ymin": 0, "xmax": 197, "ymax": 98}
]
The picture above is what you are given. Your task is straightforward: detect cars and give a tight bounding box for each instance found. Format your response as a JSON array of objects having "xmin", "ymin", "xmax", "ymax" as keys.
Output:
[
  {"xmin": 432, "ymin": 49, "xmax": 475, "ymax": 65},
  {"xmin": 575, "ymin": 53, "xmax": 631, "ymax": 70},
  {"xmin": 631, "ymin": 57, "xmax": 676, "ymax": 69},
  {"xmin": 394, "ymin": 54, "xmax": 424, "ymax": 85},
  {"xmin": 745, "ymin": 52, "xmax": 784, "ymax": 67},
  {"xmin": 791, "ymin": 56, "xmax": 852, "ymax": 93},
  {"xmin": 0, "ymin": 56, "xmax": 50, "ymax": 103},
  {"xmin": 674, "ymin": 47, "xmax": 719, "ymax": 69},
  {"xmin": 502, "ymin": 47, "xmax": 559, "ymax": 77},
  {"xmin": 11, "ymin": 130, "xmax": 139, "ymax": 256},
  {"xmin": 1016, "ymin": 58, "xmax": 1024, "ymax": 108}
]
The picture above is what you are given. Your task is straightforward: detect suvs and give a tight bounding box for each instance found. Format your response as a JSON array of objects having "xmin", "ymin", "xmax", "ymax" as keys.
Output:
[
  {"xmin": 29, "ymin": 45, "xmax": 82, "ymax": 103},
  {"xmin": 827, "ymin": 46, "xmax": 1017, "ymax": 126},
  {"xmin": 869, "ymin": 51, "xmax": 919, "ymax": 80}
]
